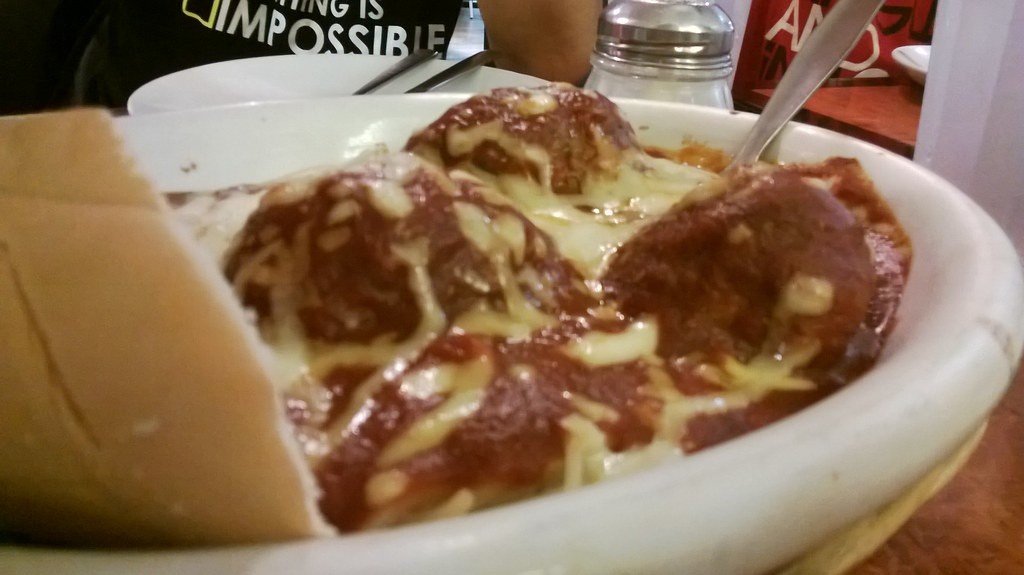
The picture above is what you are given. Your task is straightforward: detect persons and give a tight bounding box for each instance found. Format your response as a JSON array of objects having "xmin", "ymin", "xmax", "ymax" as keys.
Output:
[{"xmin": 0, "ymin": 0, "xmax": 938, "ymax": 106}]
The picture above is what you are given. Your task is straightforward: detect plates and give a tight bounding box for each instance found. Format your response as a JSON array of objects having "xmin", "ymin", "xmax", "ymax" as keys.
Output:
[
  {"xmin": 126, "ymin": 54, "xmax": 554, "ymax": 116},
  {"xmin": 891, "ymin": 45, "xmax": 932, "ymax": 86}
]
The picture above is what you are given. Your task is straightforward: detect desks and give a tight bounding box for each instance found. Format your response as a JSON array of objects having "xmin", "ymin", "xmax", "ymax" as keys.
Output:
[{"xmin": 750, "ymin": 86, "xmax": 924, "ymax": 160}]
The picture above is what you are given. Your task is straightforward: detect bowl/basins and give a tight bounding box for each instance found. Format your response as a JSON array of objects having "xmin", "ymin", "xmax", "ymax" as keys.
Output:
[{"xmin": 0, "ymin": 94, "xmax": 1024, "ymax": 575}]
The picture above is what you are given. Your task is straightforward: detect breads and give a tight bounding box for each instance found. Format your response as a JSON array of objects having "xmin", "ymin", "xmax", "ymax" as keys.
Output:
[{"xmin": 0, "ymin": 103, "xmax": 322, "ymax": 547}]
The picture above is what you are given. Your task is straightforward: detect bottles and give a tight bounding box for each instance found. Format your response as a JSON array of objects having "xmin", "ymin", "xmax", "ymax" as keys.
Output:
[
  {"xmin": 911, "ymin": 0, "xmax": 1023, "ymax": 268},
  {"xmin": 583, "ymin": 0, "xmax": 735, "ymax": 111}
]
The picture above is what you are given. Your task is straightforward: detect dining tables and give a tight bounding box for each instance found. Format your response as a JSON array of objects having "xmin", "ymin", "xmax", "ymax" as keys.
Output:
[{"xmin": 849, "ymin": 356, "xmax": 1024, "ymax": 575}]
